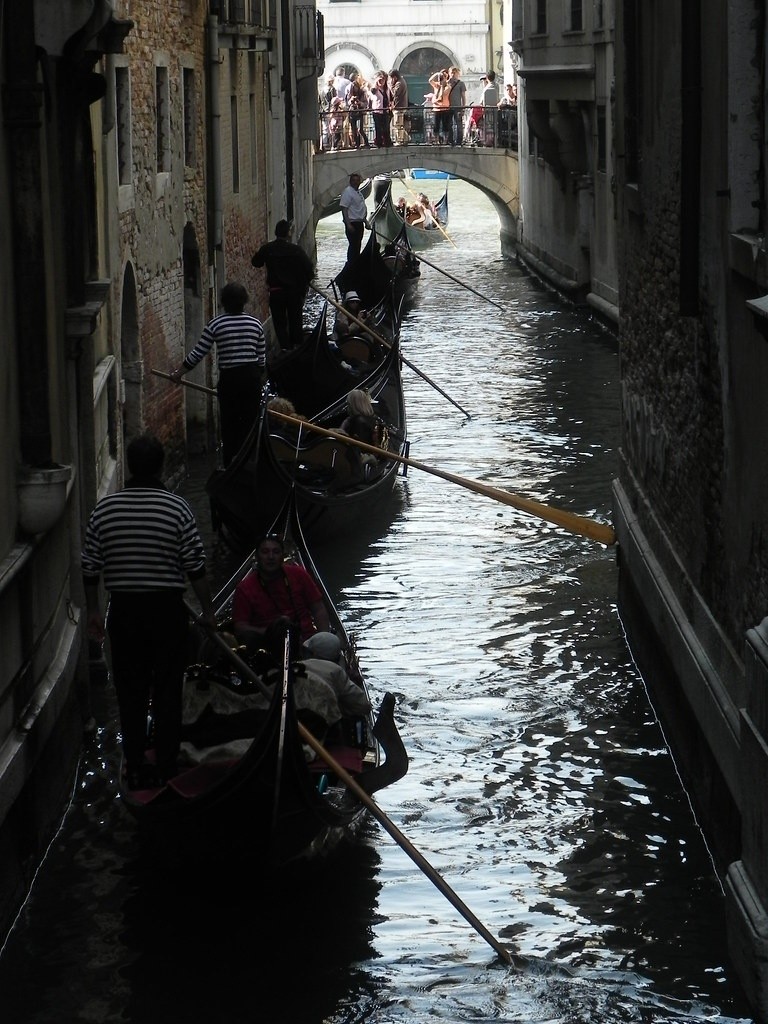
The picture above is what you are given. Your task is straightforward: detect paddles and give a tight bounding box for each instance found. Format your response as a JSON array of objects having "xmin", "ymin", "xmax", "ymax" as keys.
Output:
[
  {"xmin": 307, "ymin": 280, "xmax": 474, "ymax": 422},
  {"xmin": 181, "ymin": 600, "xmax": 513, "ymax": 964},
  {"xmin": 363, "ymin": 223, "xmax": 507, "ymax": 313},
  {"xmin": 397, "ymin": 172, "xmax": 458, "ymax": 250},
  {"xmin": 146, "ymin": 359, "xmax": 614, "ymax": 544}
]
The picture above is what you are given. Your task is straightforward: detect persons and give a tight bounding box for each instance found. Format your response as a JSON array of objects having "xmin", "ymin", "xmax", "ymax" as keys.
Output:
[
  {"xmin": 317, "ymin": 66, "xmax": 412, "ymax": 149},
  {"xmin": 168, "ymin": 283, "xmax": 267, "ymax": 471},
  {"xmin": 397, "ymin": 192, "xmax": 439, "ymax": 229},
  {"xmin": 80, "ymin": 436, "xmax": 212, "ymax": 792},
  {"xmin": 251, "ymin": 219, "xmax": 317, "ymax": 351},
  {"xmin": 374, "ymin": 244, "xmax": 397, "ymax": 257},
  {"xmin": 267, "ymin": 390, "xmax": 389, "ymax": 458},
  {"xmin": 231, "ymin": 532, "xmax": 372, "ymax": 736},
  {"xmin": 340, "ymin": 173, "xmax": 371, "ymax": 260},
  {"xmin": 334, "ymin": 291, "xmax": 380, "ymax": 350},
  {"xmin": 478, "ymin": 69, "xmax": 518, "ymax": 147},
  {"xmin": 422, "ymin": 67, "xmax": 467, "ymax": 146}
]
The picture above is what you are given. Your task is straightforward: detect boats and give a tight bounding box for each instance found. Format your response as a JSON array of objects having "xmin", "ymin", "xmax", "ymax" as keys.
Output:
[
  {"xmin": 327, "ymin": 204, "xmax": 421, "ymax": 318},
  {"xmin": 208, "ymin": 278, "xmax": 410, "ymax": 547},
  {"xmin": 367, "ymin": 174, "xmax": 448, "ymax": 250},
  {"xmin": 119, "ymin": 487, "xmax": 409, "ymax": 887}
]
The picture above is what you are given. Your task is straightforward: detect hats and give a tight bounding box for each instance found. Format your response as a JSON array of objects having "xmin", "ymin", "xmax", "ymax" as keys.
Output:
[
  {"xmin": 344, "ymin": 290, "xmax": 360, "ymax": 301},
  {"xmin": 303, "ymin": 632, "xmax": 341, "ymax": 660}
]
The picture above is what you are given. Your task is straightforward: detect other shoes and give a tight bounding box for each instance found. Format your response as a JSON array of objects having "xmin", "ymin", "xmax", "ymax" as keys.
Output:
[
  {"xmin": 127, "ymin": 762, "xmax": 164, "ymax": 791},
  {"xmin": 169, "ymin": 749, "xmax": 200, "ymax": 778}
]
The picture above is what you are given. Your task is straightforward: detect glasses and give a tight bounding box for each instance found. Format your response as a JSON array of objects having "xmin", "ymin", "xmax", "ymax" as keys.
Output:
[
  {"xmin": 264, "ymin": 532, "xmax": 278, "ymax": 541},
  {"xmin": 346, "ymin": 300, "xmax": 360, "ymax": 303}
]
